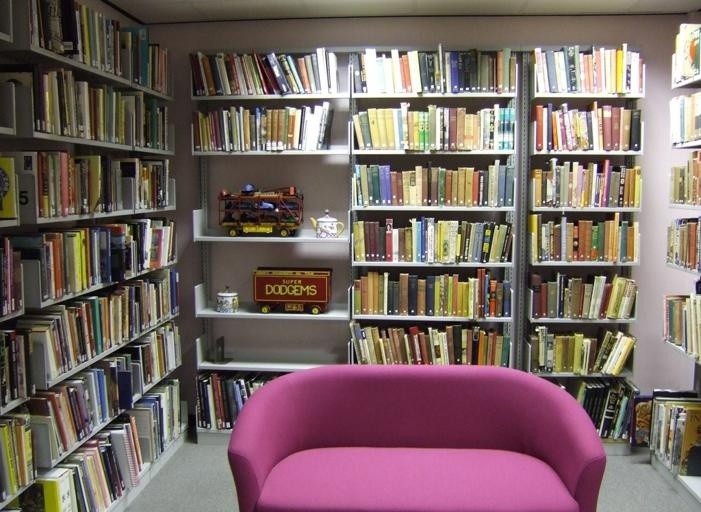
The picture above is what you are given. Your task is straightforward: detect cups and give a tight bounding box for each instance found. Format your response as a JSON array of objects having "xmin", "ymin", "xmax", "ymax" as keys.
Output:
[{"xmin": 215, "ymin": 285, "xmax": 239, "ymax": 314}]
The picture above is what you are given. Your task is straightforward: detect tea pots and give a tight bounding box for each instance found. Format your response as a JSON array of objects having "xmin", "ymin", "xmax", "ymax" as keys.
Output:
[{"xmin": 308, "ymin": 207, "xmax": 345, "ymax": 239}]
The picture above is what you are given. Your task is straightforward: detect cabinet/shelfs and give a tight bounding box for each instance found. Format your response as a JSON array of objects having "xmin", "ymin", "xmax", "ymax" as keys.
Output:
[
  {"xmin": 351, "ymin": 47, "xmax": 523, "ymax": 366},
  {"xmin": 15, "ymin": 0, "xmax": 188, "ymax": 512},
  {"xmin": 517, "ymin": 45, "xmax": 642, "ymax": 456},
  {"xmin": 0, "ymin": 0, "xmax": 31, "ymax": 512},
  {"xmin": 648, "ymin": 51, "xmax": 700, "ymax": 512},
  {"xmin": 186, "ymin": 46, "xmax": 351, "ymax": 444}
]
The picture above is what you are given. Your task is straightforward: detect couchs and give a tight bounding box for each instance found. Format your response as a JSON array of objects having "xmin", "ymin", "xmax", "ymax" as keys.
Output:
[{"xmin": 225, "ymin": 363, "xmax": 608, "ymax": 512}]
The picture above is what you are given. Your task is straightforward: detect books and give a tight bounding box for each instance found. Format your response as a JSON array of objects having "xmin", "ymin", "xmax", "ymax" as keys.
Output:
[
  {"xmin": 670, "ymin": 92, "xmax": 701, "ymax": 143},
  {"xmin": 0, "ymin": 0, "xmax": 180, "ymax": 512},
  {"xmin": 195, "ymin": 370, "xmax": 279, "ymax": 431},
  {"xmin": 349, "ymin": 43, "xmax": 515, "ymax": 367},
  {"xmin": 665, "ymin": 217, "xmax": 701, "ymax": 273},
  {"xmin": 631, "ymin": 388, "xmax": 701, "ymax": 476},
  {"xmin": 670, "ymin": 150, "xmax": 701, "ymax": 206},
  {"xmin": 662, "ymin": 295, "xmax": 701, "ymax": 360},
  {"xmin": 532, "ymin": 43, "xmax": 644, "ymax": 442},
  {"xmin": 672, "ymin": 24, "xmax": 701, "ymax": 88},
  {"xmin": 188, "ymin": 50, "xmax": 339, "ymax": 152}
]
